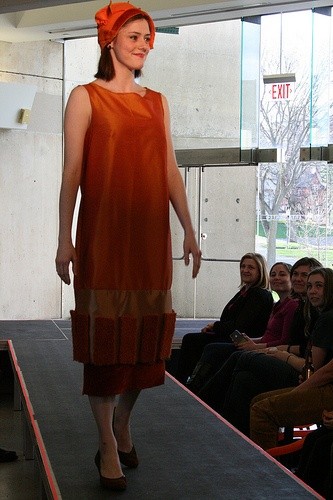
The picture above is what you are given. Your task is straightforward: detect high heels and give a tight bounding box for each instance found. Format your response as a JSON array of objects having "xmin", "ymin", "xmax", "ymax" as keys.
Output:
[
  {"xmin": 112, "ymin": 407, "xmax": 139, "ymax": 471},
  {"xmin": 95, "ymin": 451, "xmax": 127, "ymax": 495}
]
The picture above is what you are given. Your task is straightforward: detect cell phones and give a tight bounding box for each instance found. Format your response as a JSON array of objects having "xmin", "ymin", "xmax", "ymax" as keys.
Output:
[{"xmin": 230, "ymin": 330, "xmax": 247, "ymax": 345}]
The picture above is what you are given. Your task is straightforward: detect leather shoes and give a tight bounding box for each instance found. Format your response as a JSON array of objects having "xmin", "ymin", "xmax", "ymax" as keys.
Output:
[{"xmin": 0, "ymin": 449, "xmax": 19, "ymax": 463}]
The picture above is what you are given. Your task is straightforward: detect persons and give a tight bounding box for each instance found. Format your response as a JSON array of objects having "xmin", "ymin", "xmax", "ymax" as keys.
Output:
[
  {"xmin": 186, "ymin": 262, "xmax": 301, "ymax": 395},
  {"xmin": 294, "ymin": 409, "xmax": 333, "ymax": 500},
  {"xmin": 250, "ymin": 359, "xmax": 333, "ymax": 451},
  {"xmin": 55, "ymin": 2, "xmax": 201, "ymax": 491},
  {"xmin": 198, "ymin": 257, "xmax": 323, "ymax": 417},
  {"xmin": 213, "ymin": 268, "xmax": 333, "ymax": 428},
  {"xmin": 174, "ymin": 253, "xmax": 274, "ymax": 385}
]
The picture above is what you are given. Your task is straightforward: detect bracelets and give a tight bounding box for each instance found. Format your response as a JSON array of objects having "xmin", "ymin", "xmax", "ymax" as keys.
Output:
[
  {"xmin": 287, "ymin": 346, "xmax": 291, "ymax": 352},
  {"xmin": 286, "ymin": 354, "xmax": 293, "ymax": 364}
]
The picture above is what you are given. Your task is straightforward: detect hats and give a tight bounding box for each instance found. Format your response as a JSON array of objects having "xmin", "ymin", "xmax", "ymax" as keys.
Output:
[{"xmin": 94, "ymin": 0, "xmax": 155, "ymax": 51}]
